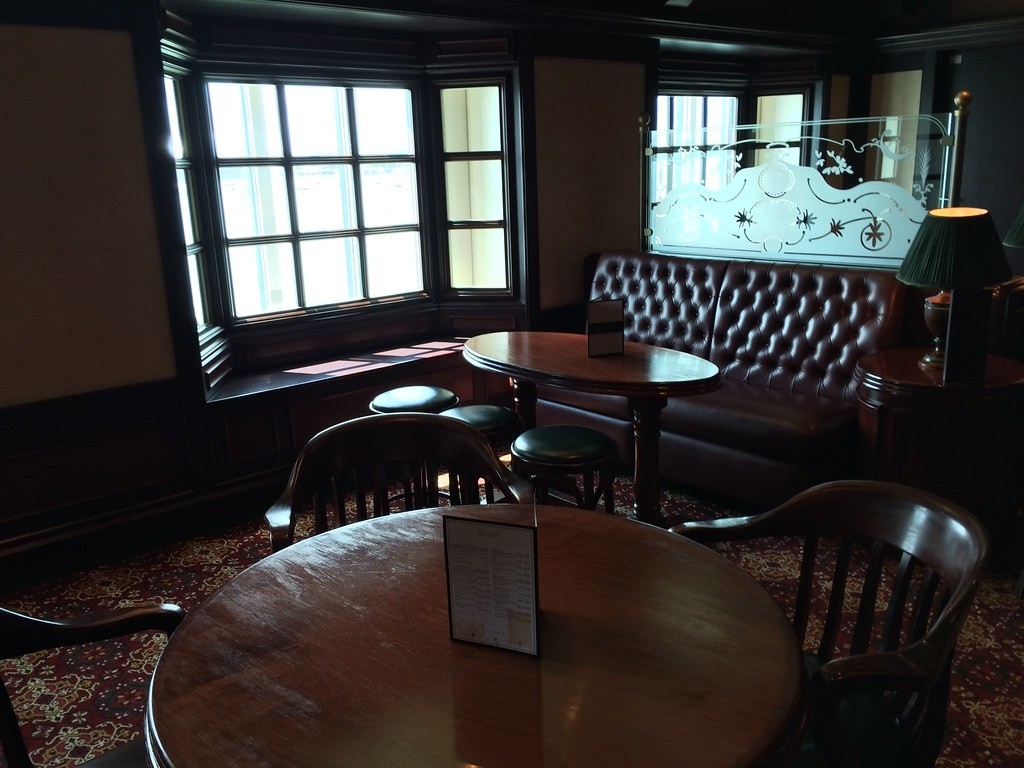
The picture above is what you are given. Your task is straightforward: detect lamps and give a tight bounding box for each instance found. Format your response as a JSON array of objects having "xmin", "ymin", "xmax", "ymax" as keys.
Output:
[{"xmin": 895, "ymin": 207, "xmax": 1014, "ymax": 372}]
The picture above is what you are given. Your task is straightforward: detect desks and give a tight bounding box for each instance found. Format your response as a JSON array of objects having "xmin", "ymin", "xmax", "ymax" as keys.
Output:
[
  {"xmin": 143, "ymin": 503, "xmax": 813, "ymax": 768},
  {"xmin": 462, "ymin": 331, "xmax": 725, "ymax": 529}
]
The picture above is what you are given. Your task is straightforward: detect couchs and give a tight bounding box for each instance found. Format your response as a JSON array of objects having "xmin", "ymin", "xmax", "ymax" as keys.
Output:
[{"xmin": 513, "ymin": 251, "xmax": 909, "ymax": 506}]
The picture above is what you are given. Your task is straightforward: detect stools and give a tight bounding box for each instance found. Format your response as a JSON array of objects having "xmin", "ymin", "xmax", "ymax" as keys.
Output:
[
  {"xmin": 368, "ymin": 384, "xmax": 459, "ymax": 515},
  {"xmin": 510, "ymin": 426, "xmax": 621, "ymax": 515},
  {"xmin": 437, "ymin": 405, "xmax": 520, "ymax": 507}
]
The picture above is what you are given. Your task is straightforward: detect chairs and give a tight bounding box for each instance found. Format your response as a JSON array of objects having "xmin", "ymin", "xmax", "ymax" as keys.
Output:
[
  {"xmin": 667, "ymin": 479, "xmax": 990, "ymax": 768},
  {"xmin": 263, "ymin": 412, "xmax": 539, "ymax": 554},
  {"xmin": 0, "ymin": 603, "xmax": 186, "ymax": 768}
]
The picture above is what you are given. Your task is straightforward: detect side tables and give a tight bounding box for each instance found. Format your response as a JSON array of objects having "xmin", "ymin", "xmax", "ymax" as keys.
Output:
[{"xmin": 848, "ymin": 347, "xmax": 1024, "ymax": 578}]
